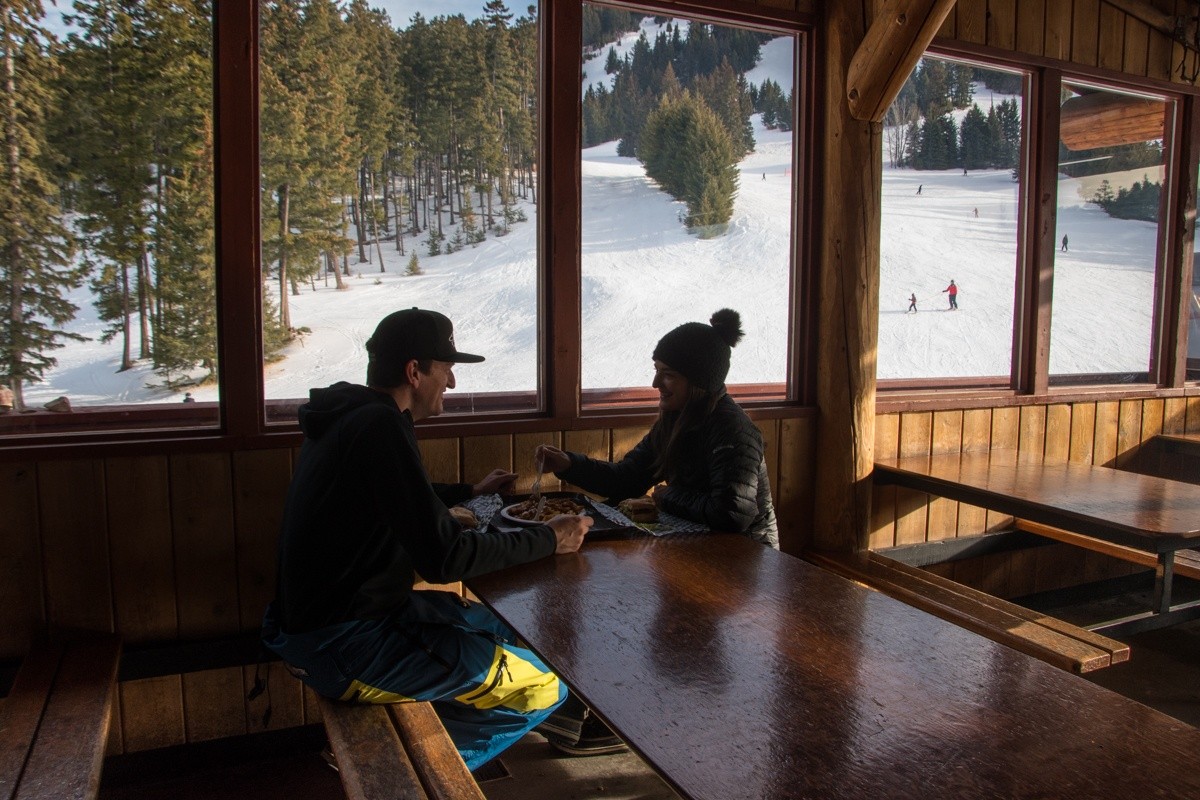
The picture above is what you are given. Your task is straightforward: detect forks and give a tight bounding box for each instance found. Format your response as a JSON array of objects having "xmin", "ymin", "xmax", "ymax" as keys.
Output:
[{"xmin": 532, "ymin": 444, "xmax": 545, "ymax": 499}]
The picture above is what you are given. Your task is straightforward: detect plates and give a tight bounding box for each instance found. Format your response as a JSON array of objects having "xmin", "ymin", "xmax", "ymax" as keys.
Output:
[{"xmin": 501, "ymin": 502, "xmax": 585, "ymax": 524}]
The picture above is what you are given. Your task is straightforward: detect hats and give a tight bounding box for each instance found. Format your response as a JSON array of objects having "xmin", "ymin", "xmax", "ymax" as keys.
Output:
[
  {"xmin": 653, "ymin": 308, "xmax": 746, "ymax": 392},
  {"xmin": 365, "ymin": 307, "xmax": 486, "ymax": 363}
]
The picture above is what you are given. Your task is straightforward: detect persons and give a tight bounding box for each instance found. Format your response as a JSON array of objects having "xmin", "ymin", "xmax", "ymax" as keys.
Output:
[
  {"xmin": 908, "ymin": 293, "xmax": 917, "ymax": 313},
  {"xmin": 535, "ymin": 308, "xmax": 780, "ymax": 552},
  {"xmin": 973, "ymin": 208, "xmax": 979, "ymax": 217},
  {"xmin": 962, "ymin": 166, "xmax": 967, "ymax": 176},
  {"xmin": 1061, "ymin": 234, "xmax": 1069, "ymax": 252},
  {"xmin": 916, "ymin": 185, "xmax": 923, "ymax": 195},
  {"xmin": 942, "ymin": 279, "xmax": 958, "ymax": 310},
  {"xmin": 278, "ymin": 307, "xmax": 593, "ymax": 772}
]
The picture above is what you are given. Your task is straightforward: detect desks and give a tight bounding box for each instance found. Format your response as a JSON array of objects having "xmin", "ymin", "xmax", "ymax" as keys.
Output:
[
  {"xmin": 1158, "ymin": 432, "xmax": 1200, "ymax": 456},
  {"xmin": 462, "ymin": 490, "xmax": 1200, "ymax": 800},
  {"xmin": 873, "ymin": 450, "xmax": 1200, "ymax": 637}
]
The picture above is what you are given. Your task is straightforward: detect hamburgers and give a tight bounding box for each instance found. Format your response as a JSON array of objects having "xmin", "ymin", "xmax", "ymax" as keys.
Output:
[
  {"xmin": 618, "ymin": 499, "xmax": 658, "ymax": 522},
  {"xmin": 450, "ymin": 507, "xmax": 479, "ymax": 527}
]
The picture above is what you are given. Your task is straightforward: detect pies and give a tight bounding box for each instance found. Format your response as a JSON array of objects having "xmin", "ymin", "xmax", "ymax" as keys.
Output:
[{"xmin": 508, "ymin": 497, "xmax": 584, "ymax": 521}]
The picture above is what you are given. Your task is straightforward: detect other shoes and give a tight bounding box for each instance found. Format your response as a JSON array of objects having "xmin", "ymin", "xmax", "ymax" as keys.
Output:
[
  {"xmin": 312, "ymin": 746, "xmax": 340, "ymax": 778},
  {"xmin": 531, "ymin": 708, "xmax": 630, "ymax": 754}
]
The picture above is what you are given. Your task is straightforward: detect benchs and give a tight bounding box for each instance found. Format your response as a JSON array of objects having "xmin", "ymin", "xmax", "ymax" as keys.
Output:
[
  {"xmin": 802, "ymin": 547, "xmax": 1130, "ymax": 672},
  {"xmin": 1018, "ymin": 517, "xmax": 1200, "ymax": 580},
  {"xmin": 314, "ymin": 690, "xmax": 489, "ymax": 800},
  {"xmin": 0, "ymin": 635, "xmax": 124, "ymax": 800}
]
